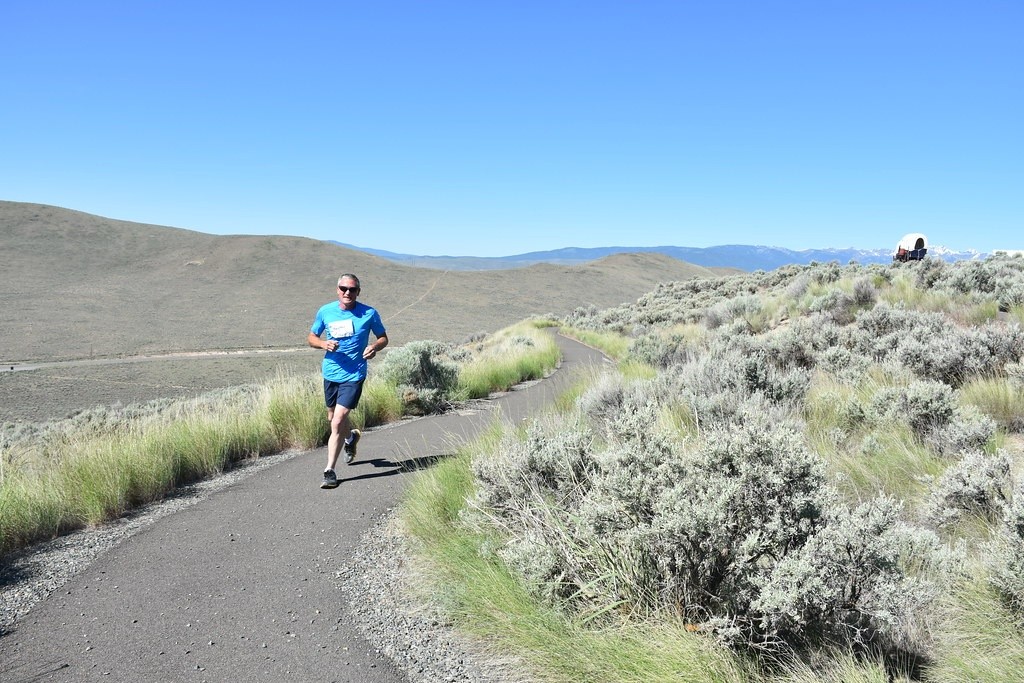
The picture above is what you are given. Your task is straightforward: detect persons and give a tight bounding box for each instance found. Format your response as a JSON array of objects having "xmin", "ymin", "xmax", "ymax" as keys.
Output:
[{"xmin": 308, "ymin": 273, "xmax": 388, "ymax": 488}]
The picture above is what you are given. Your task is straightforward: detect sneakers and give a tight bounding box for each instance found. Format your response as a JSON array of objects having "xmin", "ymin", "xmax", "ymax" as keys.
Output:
[
  {"xmin": 343, "ymin": 429, "xmax": 360, "ymax": 463},
  {"xmin": 320, "ymin": 470, "xmax": 338, "ymax": 488}
]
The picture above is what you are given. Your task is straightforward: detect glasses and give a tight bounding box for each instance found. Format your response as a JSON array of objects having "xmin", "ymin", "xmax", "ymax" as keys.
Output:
[{"xmin": 338, "ymin": 285, "xmax": 357, "ymax": 293}]
